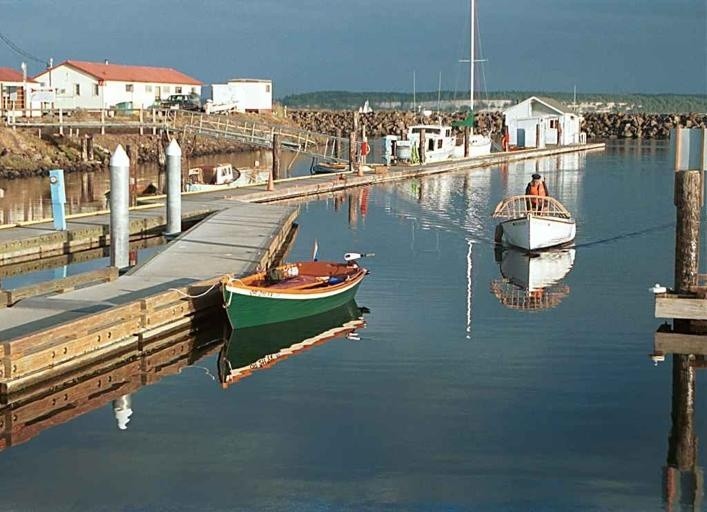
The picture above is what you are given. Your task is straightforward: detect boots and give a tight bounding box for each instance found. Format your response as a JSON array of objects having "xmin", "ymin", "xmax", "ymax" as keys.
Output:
[{"xmin": 159, "ymin": 94, "xmax": 199, "ymax": 112}]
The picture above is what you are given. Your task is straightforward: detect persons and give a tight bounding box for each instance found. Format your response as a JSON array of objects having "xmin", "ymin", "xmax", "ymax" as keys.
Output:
[{"xmin": 526, "ymin": 174, "xmax": 550, "ymax": 215}]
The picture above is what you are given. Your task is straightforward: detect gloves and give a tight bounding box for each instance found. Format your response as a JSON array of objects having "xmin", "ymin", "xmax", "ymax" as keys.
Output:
[
  {"xmin": 217, "ymin": 301, "xmax": 369, "ymax": 389},
  {"xmin": 315, "ymin": 161, "xmax": 351, "ymax": 174},
  {"xmin": 103, "ymin": 164, "xmax": 275, "ymax": 206},
  {"xmin": 493, "ymin": 213, "xmax": 579, "ymax": 251},
  {"xmin": 222, "ymin": 259, "xmax": 370, "ymax": 331},
  {"xmin": 497, "ymin": 245, "xmax": 577, "ymax": 294}
]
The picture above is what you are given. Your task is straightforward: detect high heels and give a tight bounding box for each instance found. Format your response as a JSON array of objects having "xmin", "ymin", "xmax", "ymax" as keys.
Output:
[
  {"xmin": 266, "ymin": 170, "xmax": 278, "ymax": 191},
  {"xmin": 356, "ymin": 160, "xmax": 366, "ymax": 177}
]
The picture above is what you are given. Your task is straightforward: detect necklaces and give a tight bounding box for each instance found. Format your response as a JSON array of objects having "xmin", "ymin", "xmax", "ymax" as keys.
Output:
[{"xmin": 394, "ymin": 0, "xmax": 492, "ymax": 166}]
